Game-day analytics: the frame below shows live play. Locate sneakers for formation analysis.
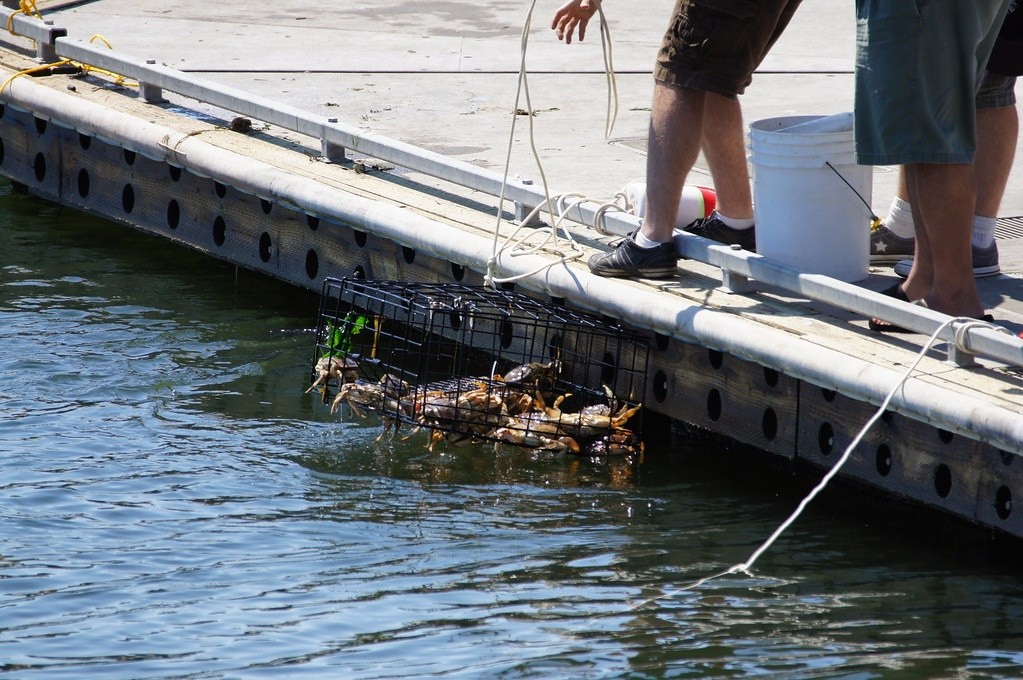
[587,226,678,277]
[869,220,916,264]
[893,239,1001,278]
[683,210,756,252]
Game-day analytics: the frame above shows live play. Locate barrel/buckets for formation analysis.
[748,113,881,283]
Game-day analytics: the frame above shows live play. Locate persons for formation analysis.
[853,0,1023,332]
[551,0,802,275]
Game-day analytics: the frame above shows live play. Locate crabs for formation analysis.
[303,357,646,462]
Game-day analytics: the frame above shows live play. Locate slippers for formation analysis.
[877,282,913,304]
[869,298,995,333]
